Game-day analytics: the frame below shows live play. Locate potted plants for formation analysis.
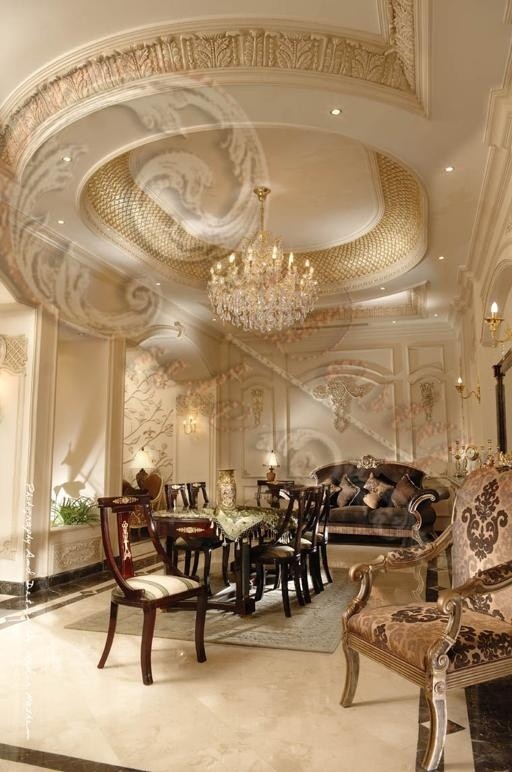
[51,495,103,576]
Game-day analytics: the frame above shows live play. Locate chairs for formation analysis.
[340,455,512,772]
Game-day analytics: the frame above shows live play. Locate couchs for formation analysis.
[297,455,440,545]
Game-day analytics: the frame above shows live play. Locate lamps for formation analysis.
[455,376,480,404]
[206,186,318,334]
[262,450,280,482]
[130,447,155,490]
[483,302,512,348]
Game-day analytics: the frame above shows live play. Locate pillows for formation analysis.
[316,472,421,510]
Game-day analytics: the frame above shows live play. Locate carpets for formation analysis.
[64,563,360,653]
